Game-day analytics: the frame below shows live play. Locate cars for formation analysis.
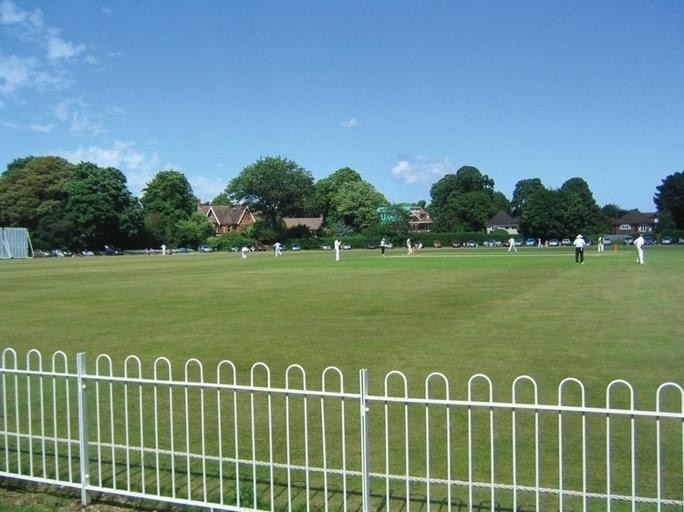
[279,244,286,251]
[290,243,300,251]
[339,242,352,250]
[318,244,332,250]
[197,243,213,253]
[367,241,378,249]
[33,243,125,257]
[414,236,684,249]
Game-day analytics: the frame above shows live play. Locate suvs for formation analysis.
[385,241,393,249]
[254,242,267,251]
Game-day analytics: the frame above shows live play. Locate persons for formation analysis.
[507,238,518,252]
[633,233,645,264]
[147,244,174,256]
[334,239,341,261]
[598,236,604,252]
[537,237,543,248]
[381,238,387,255]
[273,240,282,256]
[406,238,416,256]
[241,245,249,262]
[573,234,586,264]
[545,239,549,247]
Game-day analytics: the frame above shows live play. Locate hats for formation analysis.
[576,234,584,238]
